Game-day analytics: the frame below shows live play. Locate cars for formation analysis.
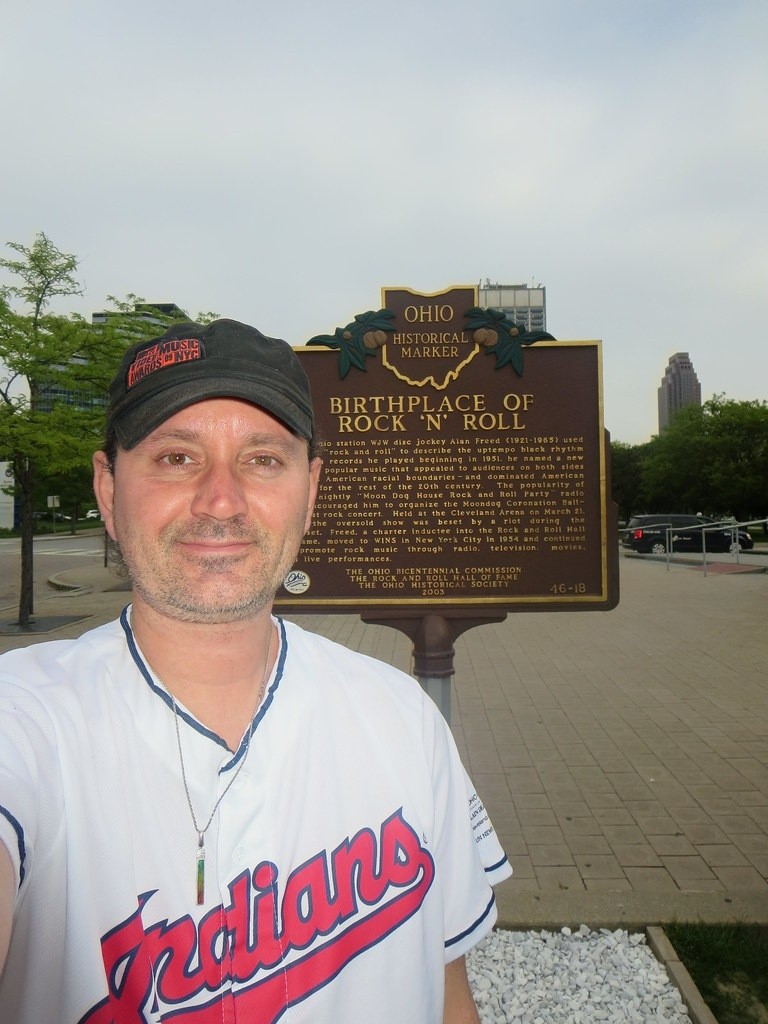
[86,510,104,521]
[32,511,71,521]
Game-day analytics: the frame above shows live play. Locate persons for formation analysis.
[0,317,514,1023]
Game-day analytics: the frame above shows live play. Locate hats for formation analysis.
[105,318,315,451]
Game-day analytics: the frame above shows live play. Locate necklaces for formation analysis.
[145,624,274,905]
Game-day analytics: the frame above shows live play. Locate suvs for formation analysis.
[622,514,754,555]
